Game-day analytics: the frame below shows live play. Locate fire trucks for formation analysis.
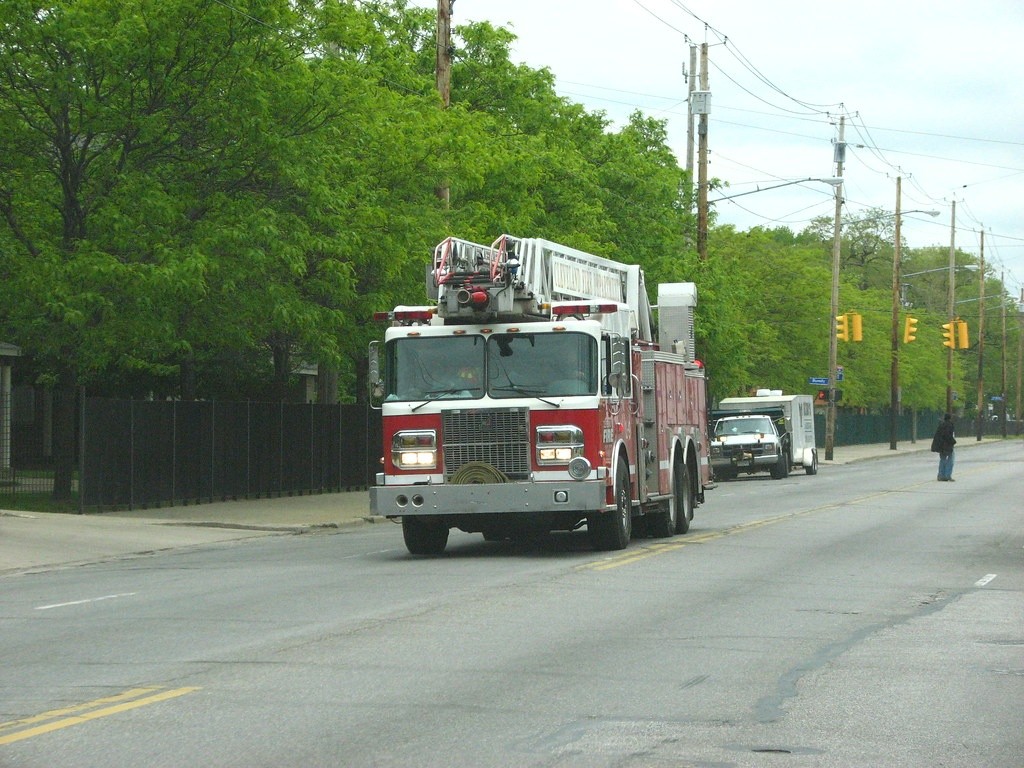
[367,234,717,557]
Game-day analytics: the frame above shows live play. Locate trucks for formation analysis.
[709,388,819,479]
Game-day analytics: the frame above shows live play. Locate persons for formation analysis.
[931,413,956,481]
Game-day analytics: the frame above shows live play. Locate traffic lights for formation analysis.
[904,314,918,344]
[832,389,843,402]
[941,322,955,349]
[817,389,827,400]
[834,313,848,342]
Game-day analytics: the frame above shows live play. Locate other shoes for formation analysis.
[947,476,955,481]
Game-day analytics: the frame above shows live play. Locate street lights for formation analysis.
[889,262,982,450]
[821,206,939,458]
[945,290,1012,413]
[693,176,843,266]
[974,297,1024,441]
